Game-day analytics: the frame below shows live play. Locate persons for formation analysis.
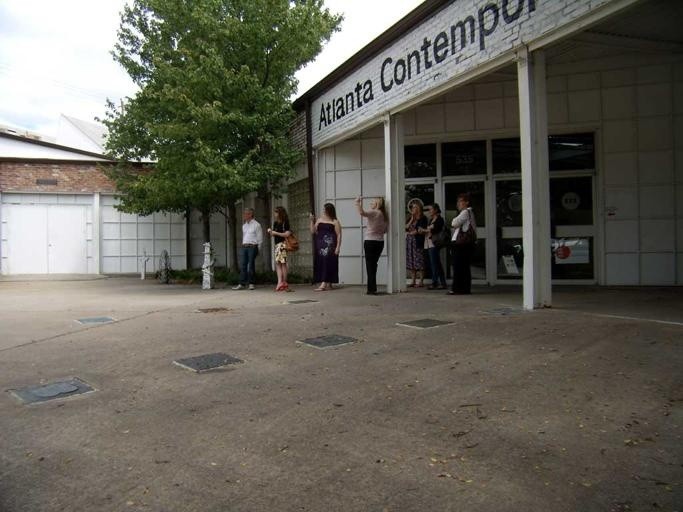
[444,196,477,296]
[307,202,341,293]
[403,200,429,289]
[266,206,292,293]
[416,201,448,291]
[351,191,388,295]
[230,207,263,291]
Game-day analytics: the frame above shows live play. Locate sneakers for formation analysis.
[408,282,469,295]
[232,284,244,290]
[314,283,333,291]
[249,284,255,290]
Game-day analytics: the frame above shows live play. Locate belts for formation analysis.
[243,244,256,248]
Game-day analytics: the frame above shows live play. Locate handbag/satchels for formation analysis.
[285,235,299,251]
[432,225,451,247]
[457,224,476,243]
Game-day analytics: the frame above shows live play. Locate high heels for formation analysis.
[272,284,288,291]
